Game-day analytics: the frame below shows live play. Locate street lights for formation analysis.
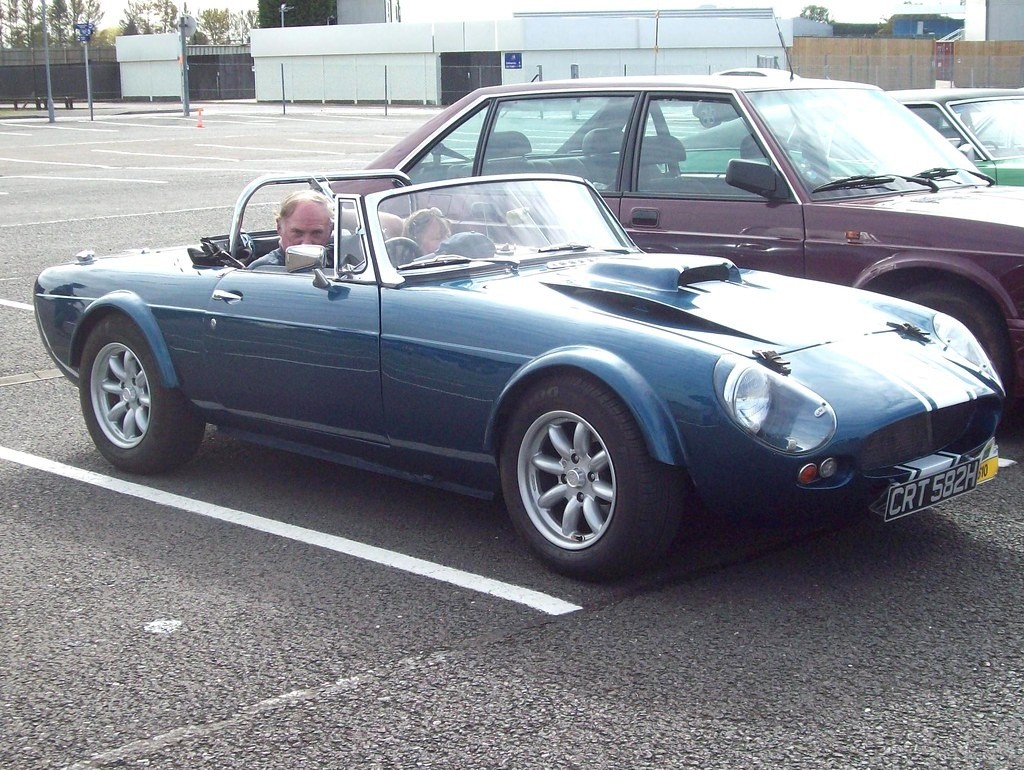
[278,4,294,28]
[327,15,334,25]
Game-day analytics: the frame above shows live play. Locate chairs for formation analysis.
[342,209,403,258]
[636,135,710,195]
[243,236,340,268]
[447,127,661,191]
[741,132,785,160]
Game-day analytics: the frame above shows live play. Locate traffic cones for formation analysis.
[197,108,204,127]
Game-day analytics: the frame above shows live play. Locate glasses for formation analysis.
[412,207,442,226]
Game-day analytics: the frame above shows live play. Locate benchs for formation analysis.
[10,97,76,108]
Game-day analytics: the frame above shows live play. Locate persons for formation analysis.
[397,208,452,266]
[245,189,405,272]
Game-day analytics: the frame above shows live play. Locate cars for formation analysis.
[663,90,1024,191]
[33,168,1018,582]
[328,74,1024,424]
